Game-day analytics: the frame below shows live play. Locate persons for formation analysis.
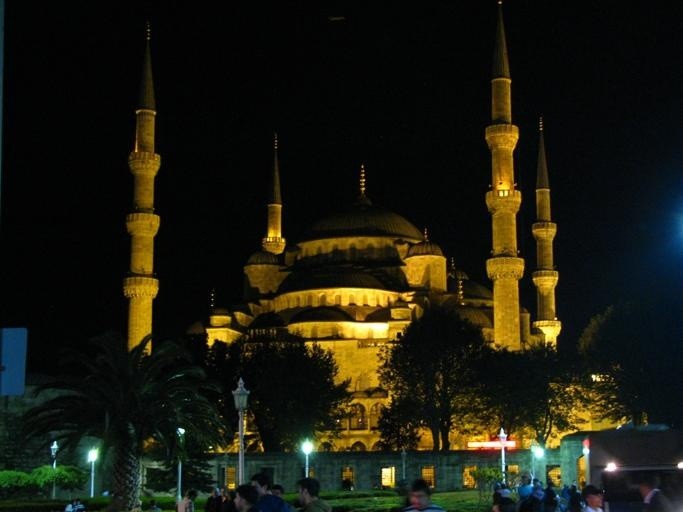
[177,490,197,512]
[297,478,332,512]
[64,498,86,512]
[146,500,161,512]
[205,473,296,512]
[492,474,604,512]
[638,476,675,512]
[402,479,446,512]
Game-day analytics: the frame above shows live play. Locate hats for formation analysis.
[583,485,608,497]
[521,471,532,480]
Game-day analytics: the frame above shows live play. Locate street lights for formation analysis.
[50,440,60,499]
[401,447,408,481]
[497,427,508,484]
[88,447,97,498]
[231,377,251,487]
[302,441,313,480]
[530,444,539,486]
[176,428,186,500]
